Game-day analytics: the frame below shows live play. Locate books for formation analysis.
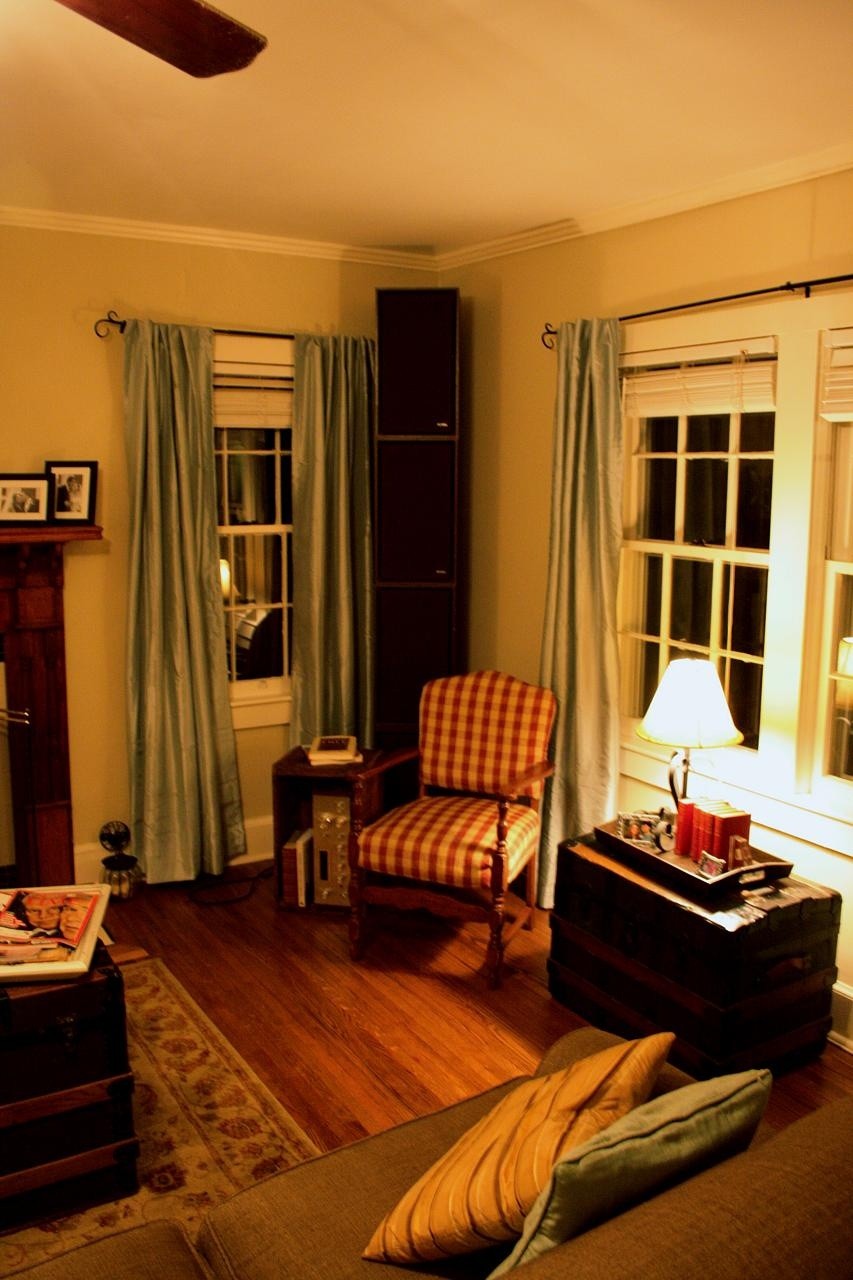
[675,798,751,872]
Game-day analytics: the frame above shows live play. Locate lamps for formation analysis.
[638,657,744,812]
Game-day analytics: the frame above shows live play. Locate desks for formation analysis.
[273,742,381,910]
[0,948,143,1230]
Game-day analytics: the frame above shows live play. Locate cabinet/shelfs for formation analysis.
[548,822,843,1082]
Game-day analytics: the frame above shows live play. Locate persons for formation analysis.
[21,894,93,939]
[56,477,76,512]
[630,822,656,841]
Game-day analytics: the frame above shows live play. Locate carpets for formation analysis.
[0,958,320,1278]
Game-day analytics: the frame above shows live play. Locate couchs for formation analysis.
[16,1028,853,1279]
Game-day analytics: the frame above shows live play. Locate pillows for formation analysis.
[364,1034,684,1264]
[489,1070,768,1280]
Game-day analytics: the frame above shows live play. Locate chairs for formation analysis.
[349,673,556,984]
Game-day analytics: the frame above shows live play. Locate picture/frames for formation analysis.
[0,884,112,984]
[0,459,99,527]
